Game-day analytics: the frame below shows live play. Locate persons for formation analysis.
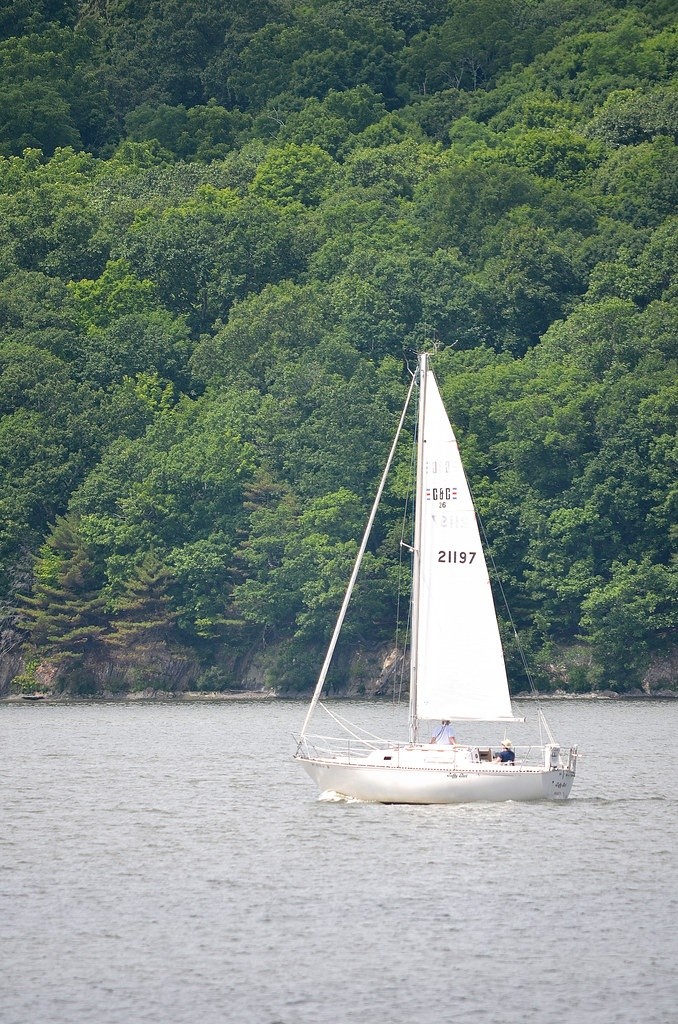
[495,740,515,766]
[430,720,457,745]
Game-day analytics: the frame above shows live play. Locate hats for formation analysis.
[502,739,513,749]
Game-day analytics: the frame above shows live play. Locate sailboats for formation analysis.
[294,352,577,806]
